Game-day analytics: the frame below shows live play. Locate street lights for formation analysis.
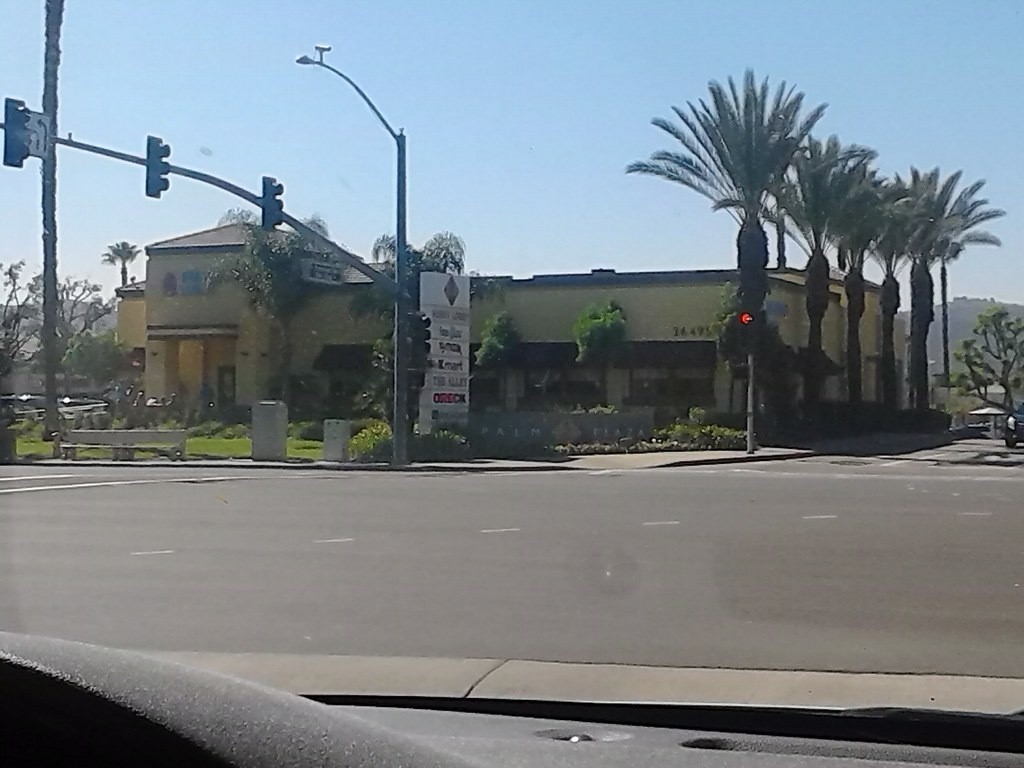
[297,55,407,465]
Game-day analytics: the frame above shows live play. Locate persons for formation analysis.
[1006,410,1020,447]
[31,372,226,439]
[952,410,963,428]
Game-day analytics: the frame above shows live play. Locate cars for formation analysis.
[1005,402,1024,447]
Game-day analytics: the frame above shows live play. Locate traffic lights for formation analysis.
[262,176,283,226]
[146,135,171,197]
[740,312,755,325]
[416,310,432,353]
[3,97,32,168]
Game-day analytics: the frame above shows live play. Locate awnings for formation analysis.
[313,342,376,370]
[761,341,845,378]
[468,340,583,368]
[615,340,721,373]
